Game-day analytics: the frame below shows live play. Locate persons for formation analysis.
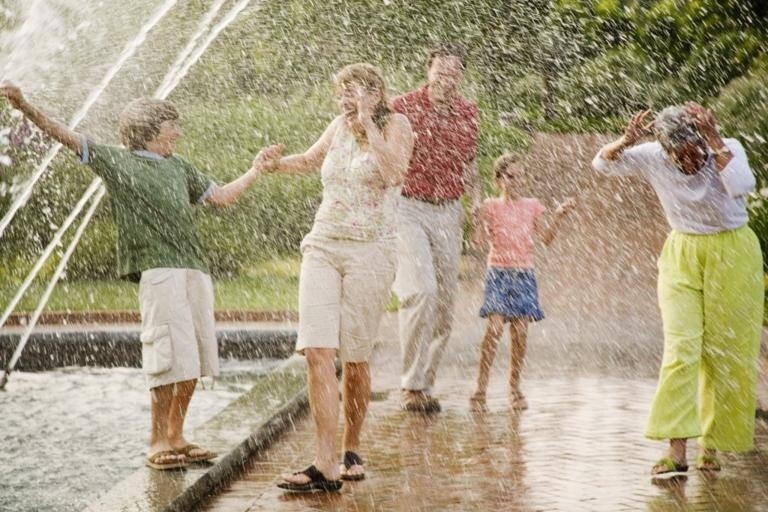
[0,79,288,470]
[468,151,577,416]
[385,46,480,413]
[252,62,414,491]
[591,102,765,483]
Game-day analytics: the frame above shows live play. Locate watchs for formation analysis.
[711,145,728,164]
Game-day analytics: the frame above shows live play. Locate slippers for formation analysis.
[339,451,365,480]
[277,465,343,493]
[650,458,689,479]
[142,444,217,469]
[696,454,721,472]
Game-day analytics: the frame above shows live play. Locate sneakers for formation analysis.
[399,389,440,414]
